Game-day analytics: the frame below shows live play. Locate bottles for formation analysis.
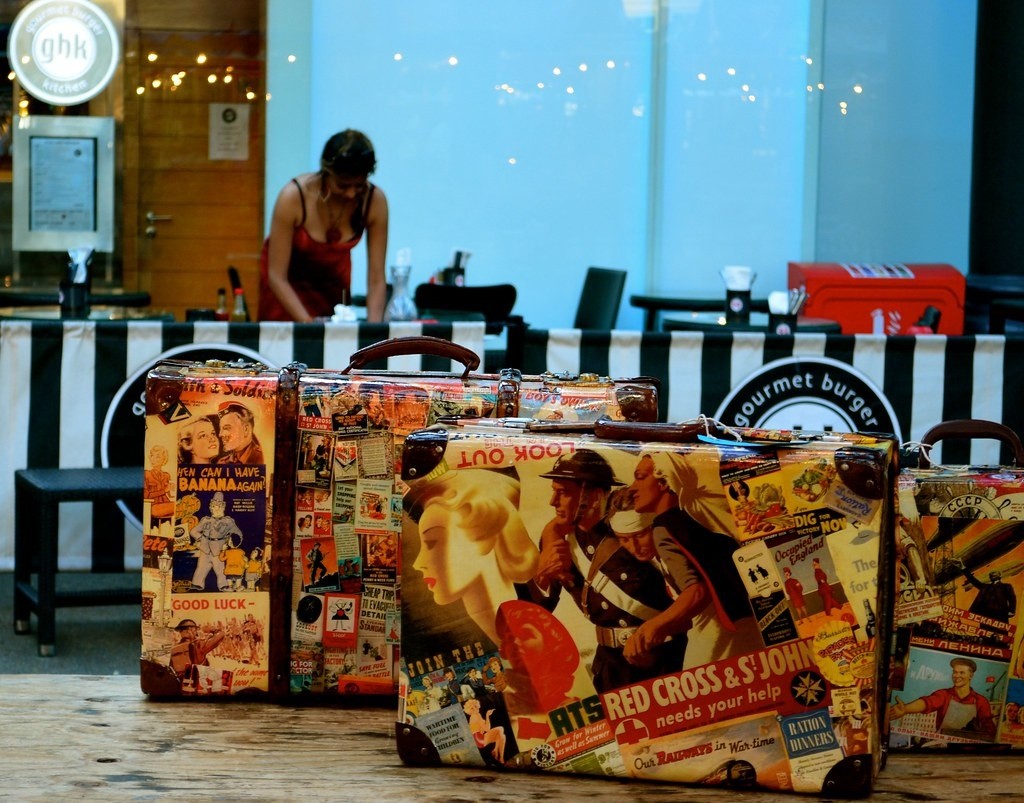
[215,288,230,321]
[233,288,245,322]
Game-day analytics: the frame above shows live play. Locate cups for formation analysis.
[726,288,751,326]
[444,268,464,288]
[769,313,797,334]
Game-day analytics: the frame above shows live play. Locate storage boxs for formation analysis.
[787,262,966,336]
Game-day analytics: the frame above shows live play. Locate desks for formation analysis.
[529,328,1023,466]
[0,318,484,571]
[0,672,1024,803]
[664,314,841,333]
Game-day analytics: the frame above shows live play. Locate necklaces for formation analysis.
[325,190,345,243]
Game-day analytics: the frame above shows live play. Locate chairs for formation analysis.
[572,267,627,329]
[630,295,771,333]
[416,284,517,320]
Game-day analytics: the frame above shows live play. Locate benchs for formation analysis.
[14,468,144,656]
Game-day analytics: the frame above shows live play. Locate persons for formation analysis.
[256,130,389,323]
